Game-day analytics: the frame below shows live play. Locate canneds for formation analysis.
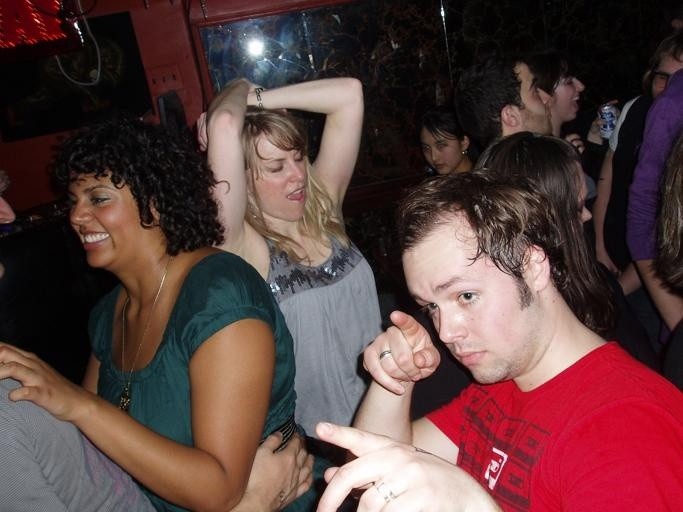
[598,105,619,138]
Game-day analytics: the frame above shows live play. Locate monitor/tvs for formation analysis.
[0,10,155,143]
